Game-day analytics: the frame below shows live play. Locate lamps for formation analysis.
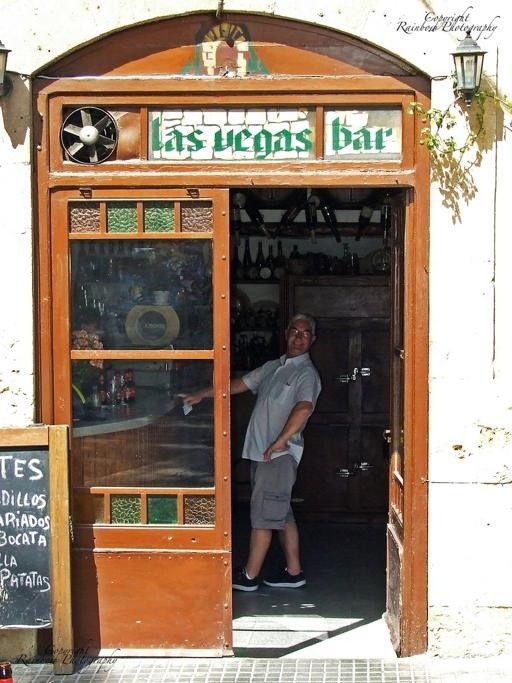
[449,27,488,109]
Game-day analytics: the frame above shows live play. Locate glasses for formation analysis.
[288,328,312,338]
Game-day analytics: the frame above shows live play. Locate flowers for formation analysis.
[70,329,107,387]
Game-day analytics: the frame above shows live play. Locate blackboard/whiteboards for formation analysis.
[0,424,72,629]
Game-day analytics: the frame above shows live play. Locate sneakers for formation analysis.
[263,568,307,588]
[233,567,259,592]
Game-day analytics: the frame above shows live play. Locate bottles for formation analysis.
[77,240,130,282]
[230,203,243,247]
[380,203,391,245]
[90,368,136,410]
[272,201,303,240]
[232,238,300,281]
[321,205,342,243]
[304,200,318,245]
[244,204,272,240]
[355,203,376,242]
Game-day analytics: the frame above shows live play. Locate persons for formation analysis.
[72,305,116,393]
[175,312,324,592]
